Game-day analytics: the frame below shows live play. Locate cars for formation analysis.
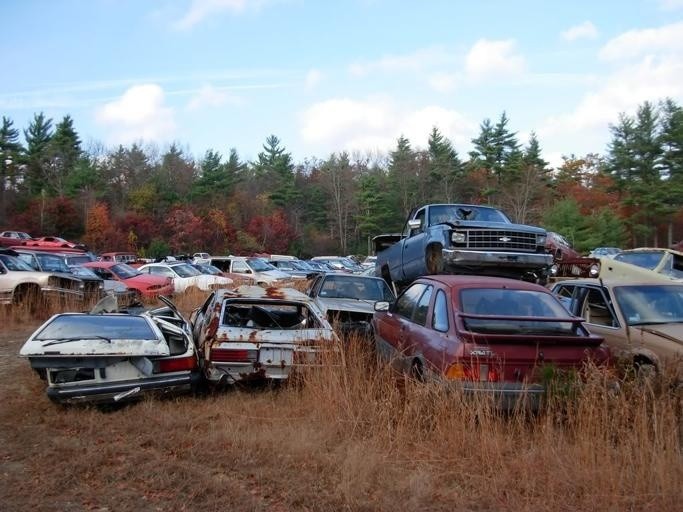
[550,276,683,397]
[370,271,620,418]
[309,271,398,345]
[597,245,683,282]
[588,247,624,258]
[189,283,346,389]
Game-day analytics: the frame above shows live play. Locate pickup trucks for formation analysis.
[373,203,555,292]
